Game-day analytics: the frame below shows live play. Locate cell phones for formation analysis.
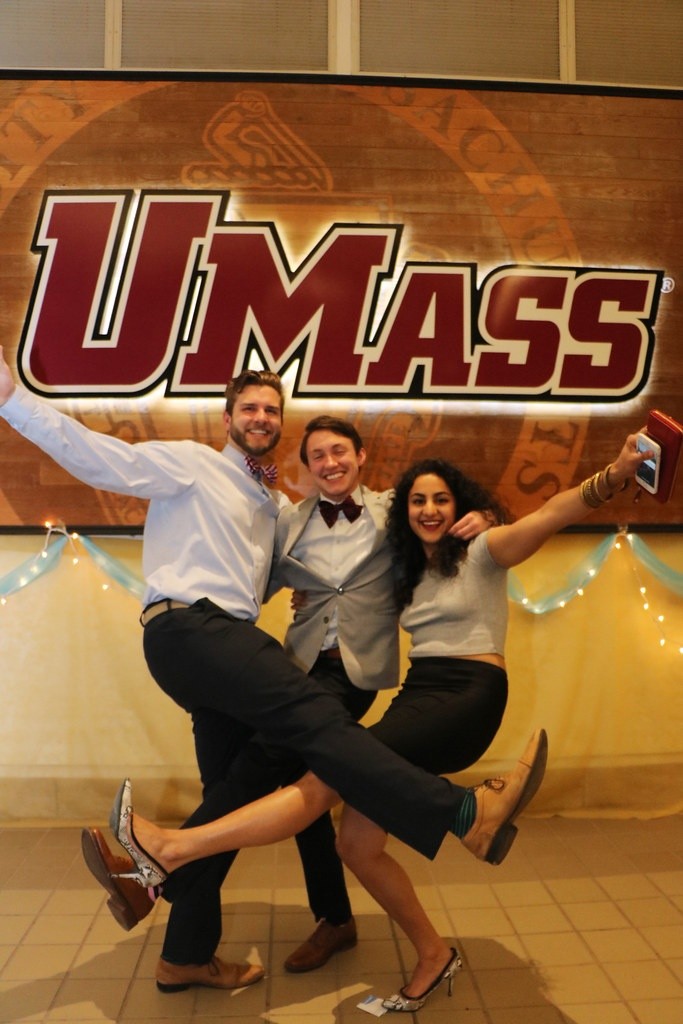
[635,433,661,494]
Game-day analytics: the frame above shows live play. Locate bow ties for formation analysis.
[319,495,363,529]
[247,455,277,484]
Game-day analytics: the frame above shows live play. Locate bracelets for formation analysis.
[579,463,630,511]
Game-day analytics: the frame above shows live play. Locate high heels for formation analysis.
[382,947,463,1012]
[108,777,168,889]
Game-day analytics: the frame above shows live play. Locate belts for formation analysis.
[142,600,190,626]
[319,646,342,659]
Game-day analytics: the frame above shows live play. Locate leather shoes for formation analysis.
[461,727,548,865]
[82,826,155,931]
[284,916,357,972]
[156,955,265,994]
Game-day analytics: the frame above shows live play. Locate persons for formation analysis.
[0,346,548,994]
[110,426,654,1010]
